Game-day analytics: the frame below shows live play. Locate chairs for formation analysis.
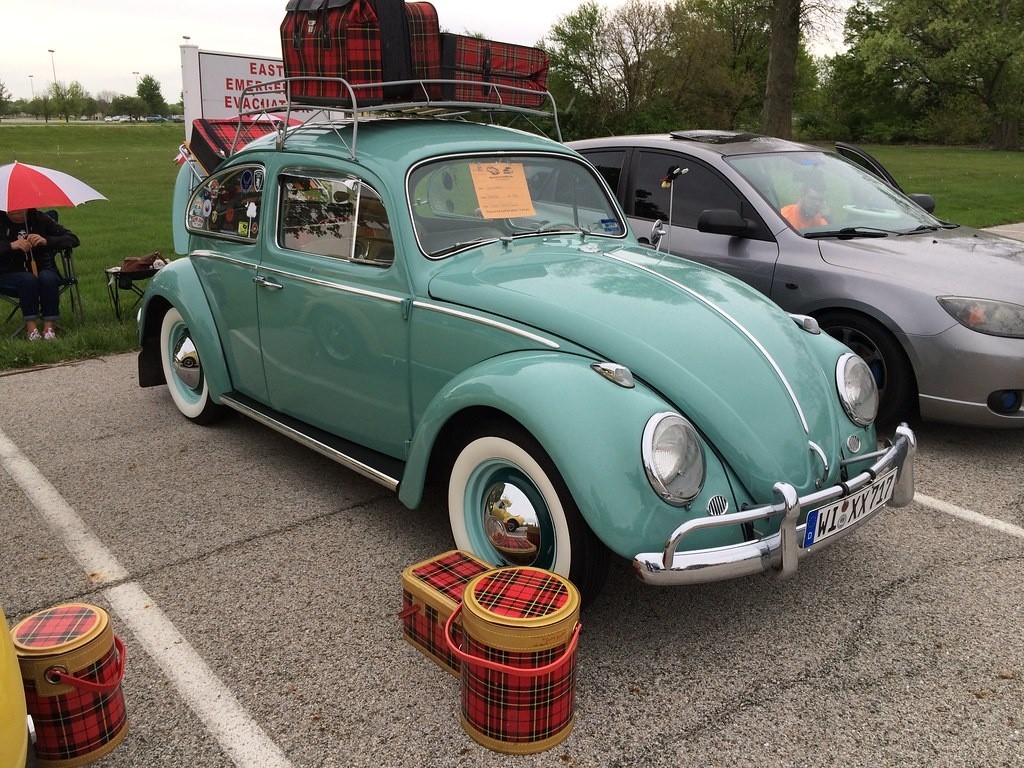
[0,210,83,338]
[422,228,506,256]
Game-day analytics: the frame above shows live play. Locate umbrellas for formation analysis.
[0,159,110,277]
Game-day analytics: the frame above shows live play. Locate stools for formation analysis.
[104,258,170,326]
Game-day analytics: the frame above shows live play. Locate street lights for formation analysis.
[28,75,36,100]
[132,72,140,88]
[48,49,57,85]
[183,35,190,45]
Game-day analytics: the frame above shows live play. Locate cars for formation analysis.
[133,76,916,614]
[476,129,1024,433]
[112,115,185,123]
[104,116,112,122]
[80,116,88,121]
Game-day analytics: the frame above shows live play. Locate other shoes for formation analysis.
[42,328,56,340]
[27,328,42,340]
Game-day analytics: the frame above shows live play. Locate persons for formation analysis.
[780,178,831,229]
[0,208,81,341]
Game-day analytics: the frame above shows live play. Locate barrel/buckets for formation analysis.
[444,565,581,755]
[11,602,130,768]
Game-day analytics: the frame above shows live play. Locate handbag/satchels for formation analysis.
[189,117,289,173]
[279,0,443,107]
[439,30,549,105]
[120,252,168,272]
